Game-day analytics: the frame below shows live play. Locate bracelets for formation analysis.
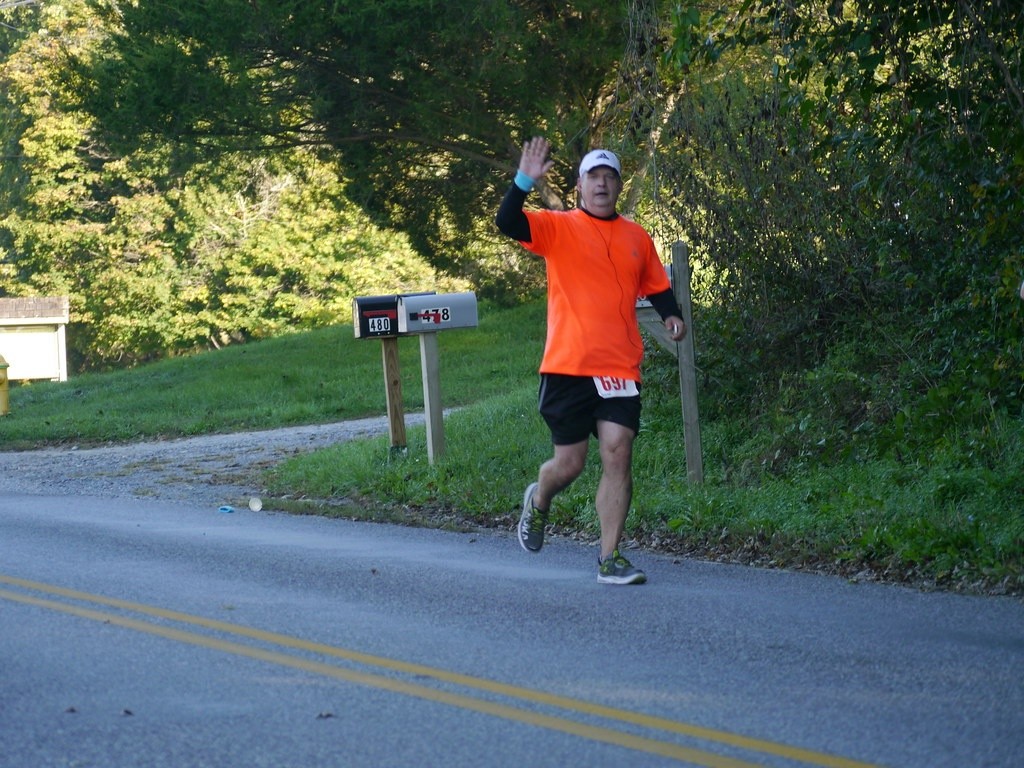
[514,170,538,194]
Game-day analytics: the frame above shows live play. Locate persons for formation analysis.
[495,137,687,585]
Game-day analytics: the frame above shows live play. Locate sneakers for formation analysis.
[597,549,647,585]
[518,482,551,553]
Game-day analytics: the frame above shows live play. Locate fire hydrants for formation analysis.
[0,352,10,416]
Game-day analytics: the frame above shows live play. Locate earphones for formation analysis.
[579,183,581,188]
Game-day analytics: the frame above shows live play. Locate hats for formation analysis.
[579,149,622,181]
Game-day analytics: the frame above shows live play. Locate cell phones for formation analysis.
[674,324,678,335]
